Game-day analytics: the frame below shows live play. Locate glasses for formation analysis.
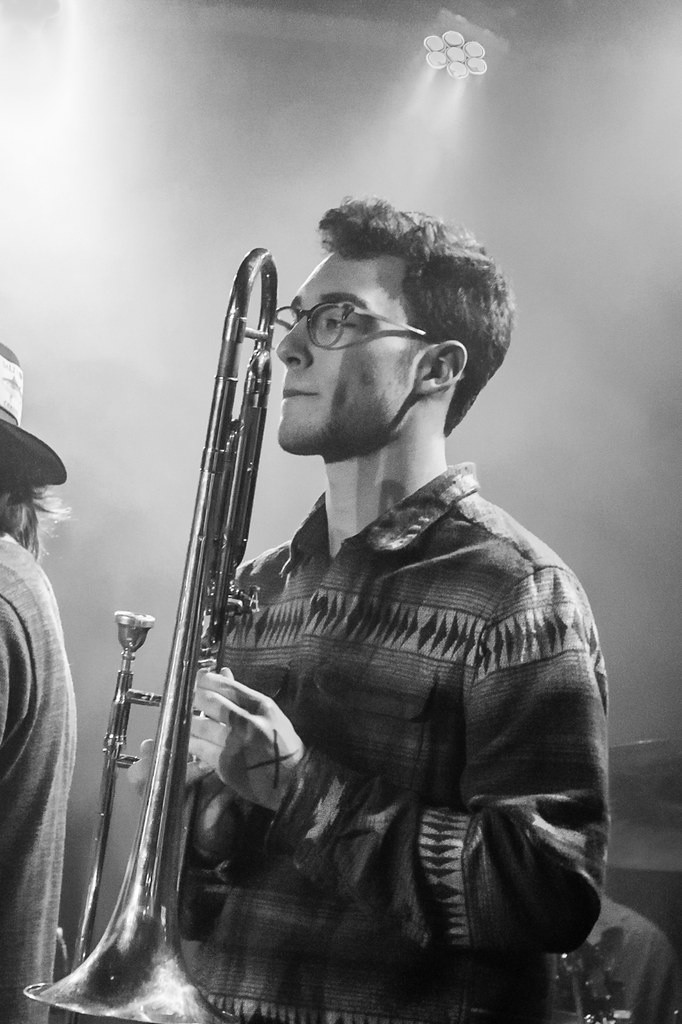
[267,302,434,351]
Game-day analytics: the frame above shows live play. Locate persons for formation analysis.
[0,419,69,1023]
[127,208,609,1023]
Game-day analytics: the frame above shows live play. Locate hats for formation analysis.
[0,341,68,488]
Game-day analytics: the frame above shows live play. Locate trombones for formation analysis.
[22,245,278,1024]
